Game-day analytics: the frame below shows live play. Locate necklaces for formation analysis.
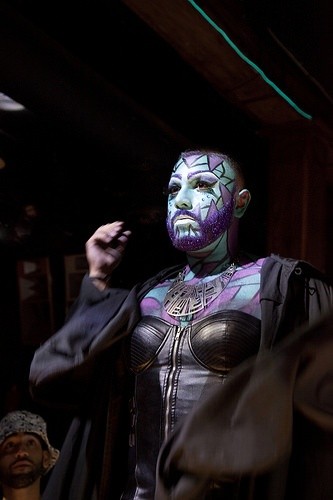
[163,260,237,318]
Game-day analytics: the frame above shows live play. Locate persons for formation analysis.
[29,149,333,498]
[0,412,62,500]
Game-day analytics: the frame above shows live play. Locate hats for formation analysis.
[0,410,58,476]
